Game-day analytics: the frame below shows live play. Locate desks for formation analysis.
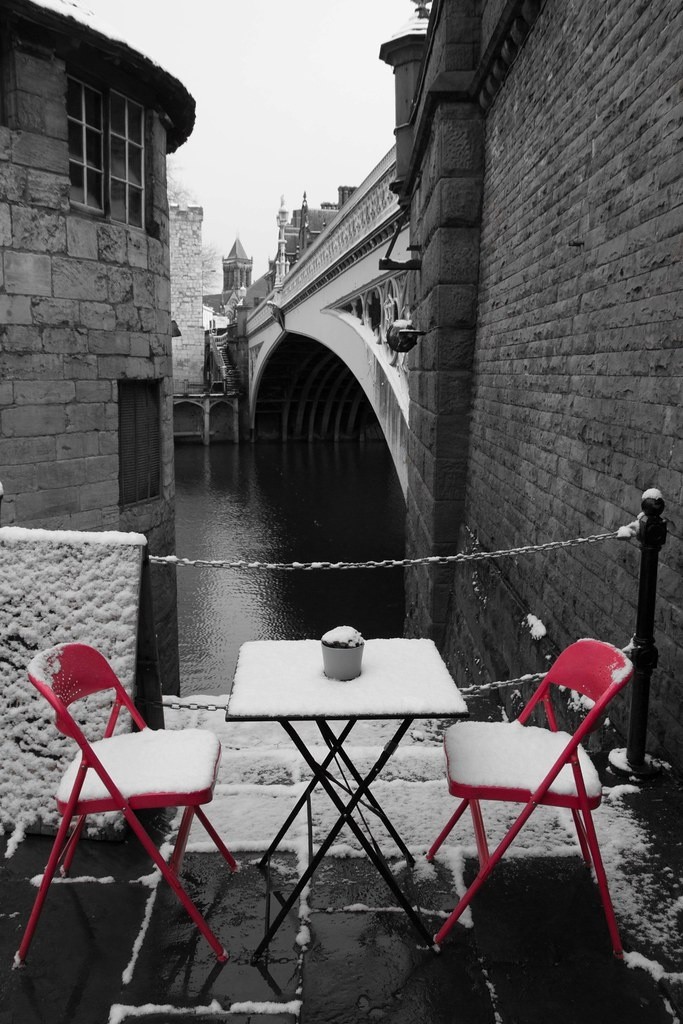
[225,639,468,966]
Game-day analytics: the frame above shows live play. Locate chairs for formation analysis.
[426,638,635,961]
[12,643,241,967]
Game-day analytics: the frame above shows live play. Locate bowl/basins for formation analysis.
[322,642,363,679]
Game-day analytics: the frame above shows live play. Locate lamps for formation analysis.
[387,324,428,353]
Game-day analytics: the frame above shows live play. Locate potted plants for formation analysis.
[321,625,365,682]
